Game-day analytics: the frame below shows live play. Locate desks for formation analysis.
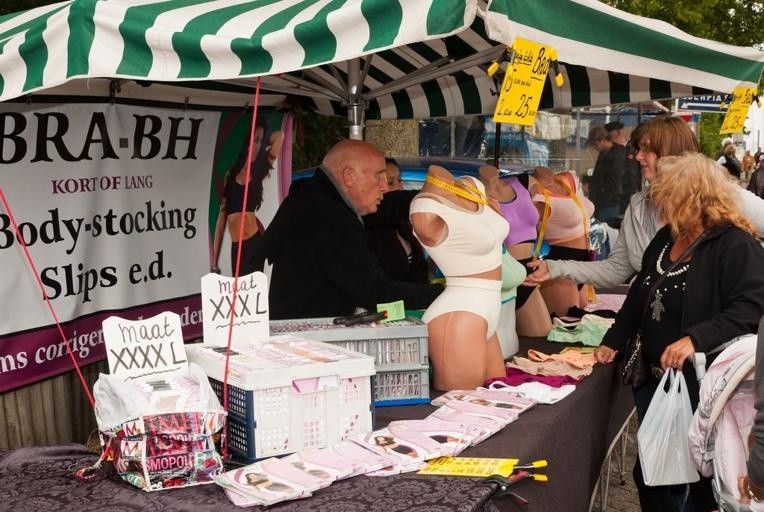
[1,278,640,512]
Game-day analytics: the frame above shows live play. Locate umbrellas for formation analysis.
[0,0,764,141]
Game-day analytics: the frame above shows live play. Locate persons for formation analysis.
[376,435,419,460]
[598,153,764,512]
[471,398,522,411]
[717,147,741,180]
[381,158,429,282]
[714,141,732,160]
[606,122,625,143]
[246,472,292,495]
[410,166,508,389]
[753,147,763,161]
[523,115,699,287]
[526,166,592,311]
[214,118,285,276]
[739,295,764,511]
[743,150,755,178]
[588,127,625,226]
[432,435,467,445]
[748,162,764,199]
[480,163,551,336]
[262,140,446,319]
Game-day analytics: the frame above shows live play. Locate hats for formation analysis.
[606,121,622,130]
[582,127,610,147]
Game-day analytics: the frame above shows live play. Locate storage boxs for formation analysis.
[184,335,374,471]
[269,318,432,407]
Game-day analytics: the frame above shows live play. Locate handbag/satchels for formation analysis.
[622,228,713,388]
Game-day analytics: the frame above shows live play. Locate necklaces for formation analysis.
[656,239,690,277]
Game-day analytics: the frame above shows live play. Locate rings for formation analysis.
[671,360,677,365]
